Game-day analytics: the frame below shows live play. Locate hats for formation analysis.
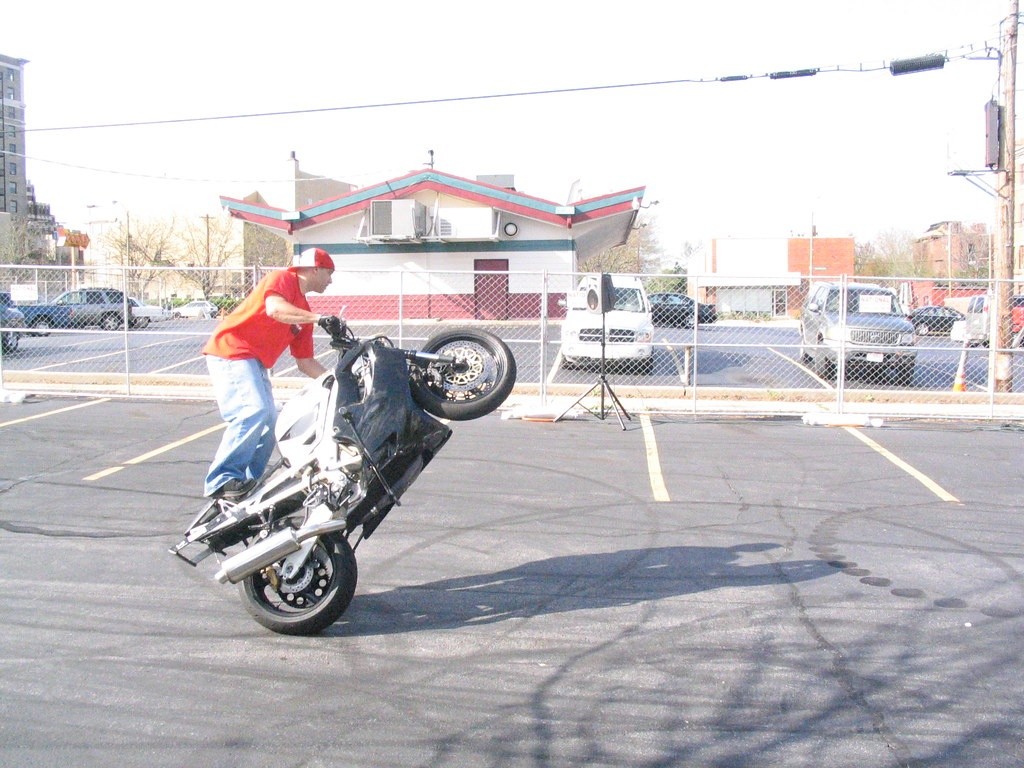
[287,247,335,270]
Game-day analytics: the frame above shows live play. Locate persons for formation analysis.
[202,248,347,498]
[1012,298,1024,348]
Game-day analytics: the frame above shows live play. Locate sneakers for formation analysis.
[209,478,257,498]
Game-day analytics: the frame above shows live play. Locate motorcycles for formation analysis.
[168,302,518,635]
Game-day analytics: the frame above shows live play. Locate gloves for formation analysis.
[318,315,342,335]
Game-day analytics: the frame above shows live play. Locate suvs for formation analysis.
[53,287,132,331]
[798,281,915,382]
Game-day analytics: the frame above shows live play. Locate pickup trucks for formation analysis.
[3,292,74,337]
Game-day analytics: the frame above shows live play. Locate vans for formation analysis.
[965,294,1024,349]
[559,273,654,376]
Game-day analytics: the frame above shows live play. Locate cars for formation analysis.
[647,294,717,329]
[128,298,169,329]
[0,303,24,356]
[171,300,218,319]
[907,306,966,336]
